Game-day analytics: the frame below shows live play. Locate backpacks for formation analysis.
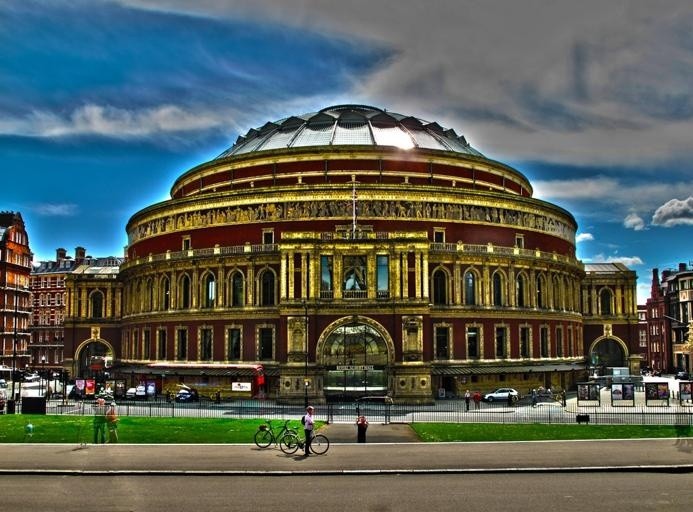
[301,416,305,425]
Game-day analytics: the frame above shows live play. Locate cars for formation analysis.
[0,377,8,410]
[24,373,40,382]
[71,381,200,404]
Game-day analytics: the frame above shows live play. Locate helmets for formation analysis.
[306,406,314,410]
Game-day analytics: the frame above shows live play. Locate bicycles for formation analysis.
[279,423,329,456]
[253,419,298,448]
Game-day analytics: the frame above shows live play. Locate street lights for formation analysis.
[52,372,59,398]
[297,297,310,407]
[10,284,29,400]
[40,354,44,395]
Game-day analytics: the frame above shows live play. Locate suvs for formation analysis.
[674,371,689,380]
[336,395,395,416]
[481,386,520,402]
